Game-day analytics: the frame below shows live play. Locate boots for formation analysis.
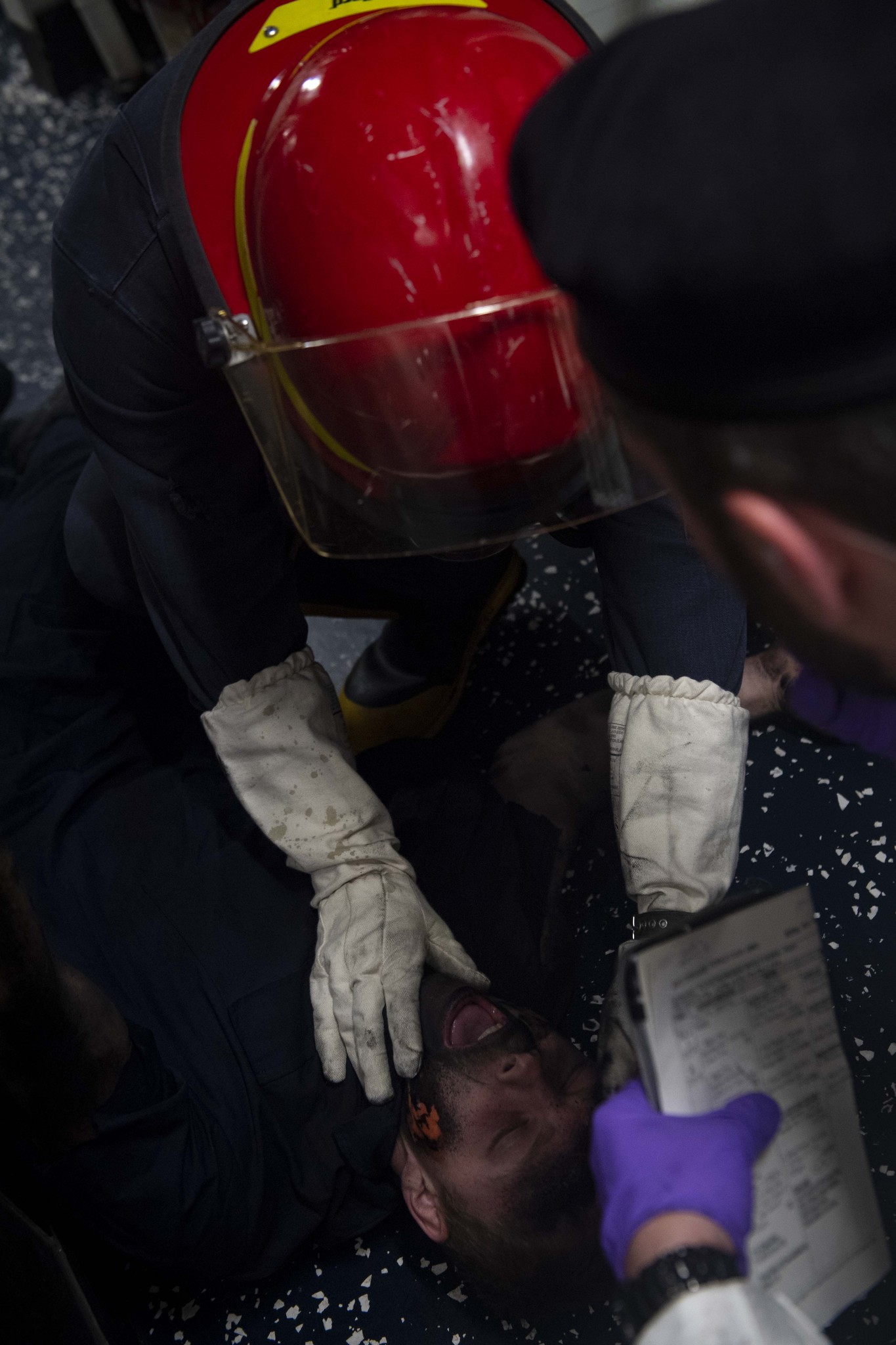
[300,553,531,757]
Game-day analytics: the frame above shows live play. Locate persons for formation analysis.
[505,0,895,1345]
[1,367,605,1344]
[45,0,750,1097]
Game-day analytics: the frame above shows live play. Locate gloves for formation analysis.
[198,643,494,1104]
[786,669,896,763]
[590,1075,780,1280]
[602,669,752,1096]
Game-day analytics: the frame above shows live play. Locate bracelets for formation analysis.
[608,1247,746,1344]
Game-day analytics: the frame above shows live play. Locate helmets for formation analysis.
[159,1,665,561]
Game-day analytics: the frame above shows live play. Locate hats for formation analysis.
[506,2,896,423]
